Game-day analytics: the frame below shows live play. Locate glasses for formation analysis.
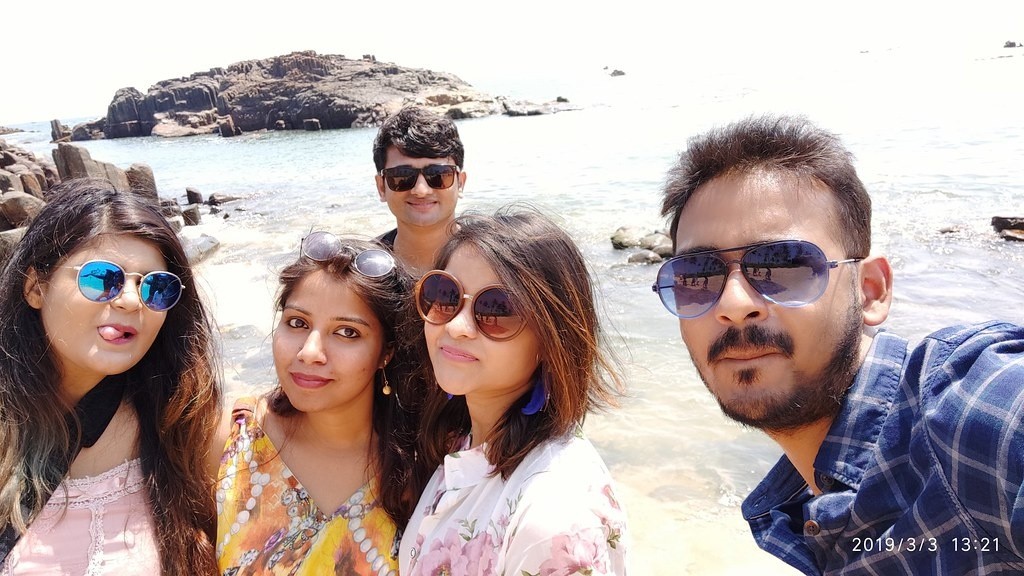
[48,260,186,313]
[415,270,534,342]
[301,231,398,278]
[651,239,862,318]
[378,165,459,192]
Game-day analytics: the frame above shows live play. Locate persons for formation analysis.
[653,112,1024,576]
[1,178,225,576]
[396,201,636,576]
[366,104,469,526]
[213,231,457,575]
